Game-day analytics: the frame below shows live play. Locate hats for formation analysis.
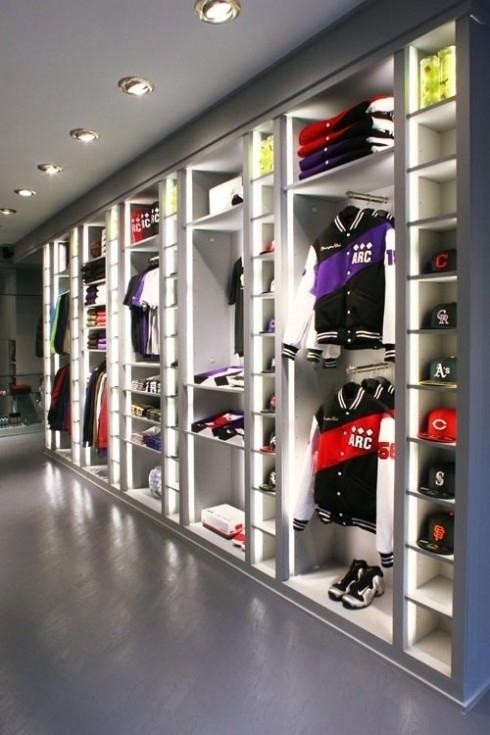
[419,407,457,443]
[418,512,453,554]
[259,240,277,491]
[420,303,456,330]
[420,358,457,387]
[419,463,454,499]
[423,251,455,274]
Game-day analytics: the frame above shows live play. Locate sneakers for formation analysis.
[342,567,384,610]
[329,559,367,601]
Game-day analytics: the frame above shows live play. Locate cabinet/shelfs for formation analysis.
[42,5,483,709]
